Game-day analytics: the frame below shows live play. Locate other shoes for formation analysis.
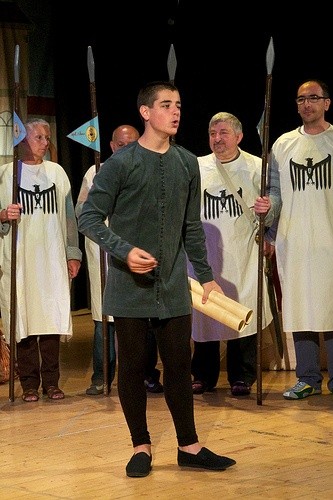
[125,452,152,477]
[177,446,236,470]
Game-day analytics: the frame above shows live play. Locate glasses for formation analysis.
[296,96,329,105]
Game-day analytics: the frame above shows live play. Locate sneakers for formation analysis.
[145,381,160,394]
[192,380,210,394]
[231,381,252,396]
[283,381,322,399]
[86,379,110,394]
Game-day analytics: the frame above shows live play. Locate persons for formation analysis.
[183,112,268,397]
[79,82,236,477]
[255,80,333,400]
[75,125,163,395]
[0,117,82,402]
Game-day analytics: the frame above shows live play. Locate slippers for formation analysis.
[22,388,40,402]
[46,386,65,399]
